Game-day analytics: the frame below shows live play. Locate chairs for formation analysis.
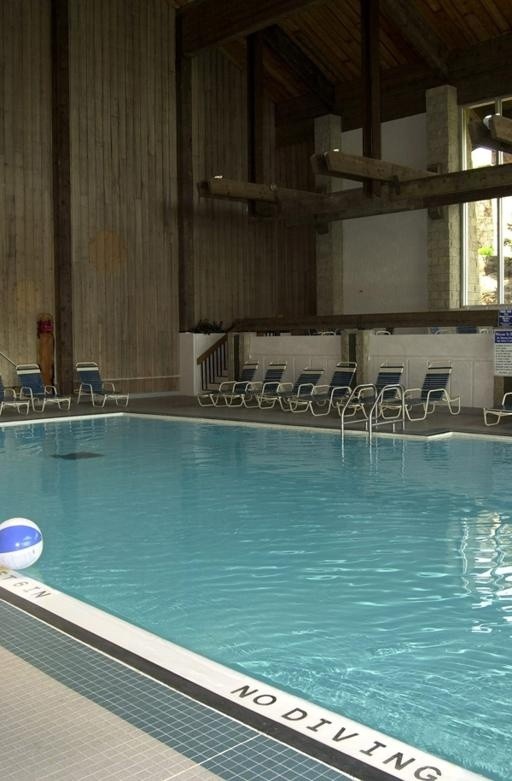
[196,357,464,428]
[74,361,132,410]
[15,362,73,414]
[0,373,30,419]
[481,391,512,428]
